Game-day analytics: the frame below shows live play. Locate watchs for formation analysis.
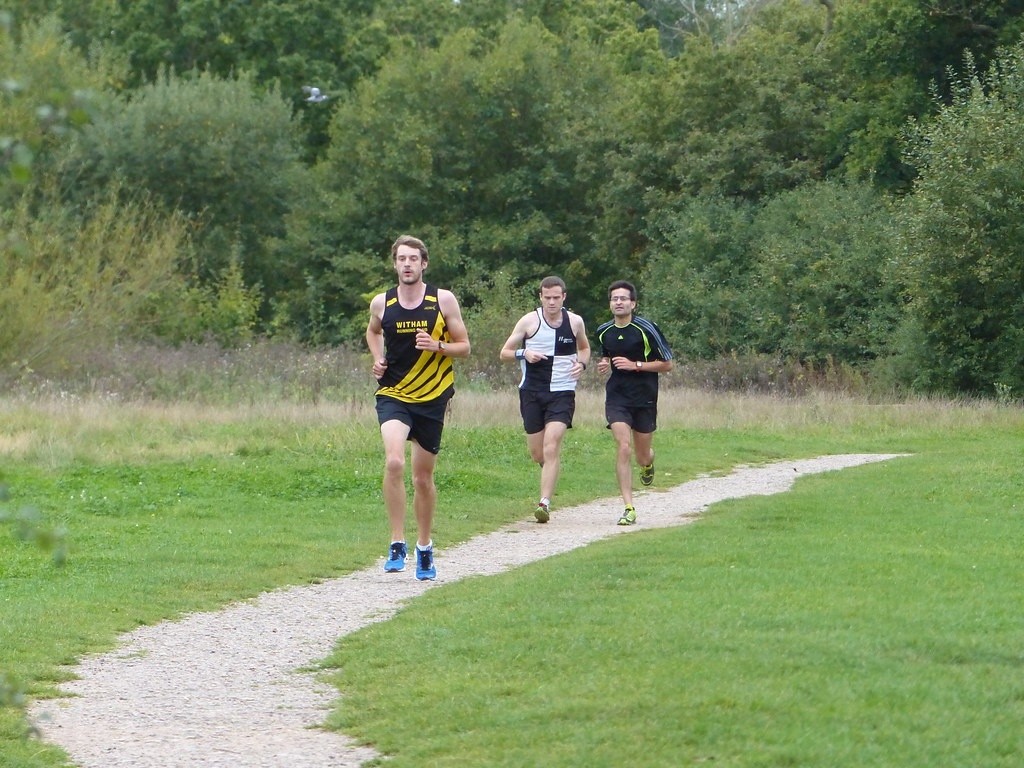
[439,340,447,355]
[636,361,643,371]
[578,362,586,371]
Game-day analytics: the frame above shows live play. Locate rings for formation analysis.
[601,369,604,373]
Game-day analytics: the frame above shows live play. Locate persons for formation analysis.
[363,233,471,581]
[595,280,674,526]
[499,275,591,522]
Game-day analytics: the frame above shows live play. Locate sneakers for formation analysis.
[535,504,549,523]
[617,505,636,525]
[384,542,409,572]
[415,548,437,580]
[640,464,654,486]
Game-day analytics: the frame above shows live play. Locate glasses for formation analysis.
[609,296,631,301]
